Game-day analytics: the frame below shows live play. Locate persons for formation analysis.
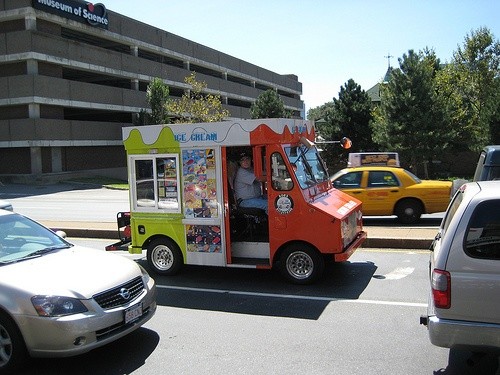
[234,151,287,218]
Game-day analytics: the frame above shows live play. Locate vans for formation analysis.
[474,145,500,181]
[420,181,499,349]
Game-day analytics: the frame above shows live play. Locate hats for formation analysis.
[237,151,249,160]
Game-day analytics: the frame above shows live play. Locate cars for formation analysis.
[0,204,159,375]
[329,166,453,224]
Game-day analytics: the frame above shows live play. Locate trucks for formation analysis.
[106,116,368,286]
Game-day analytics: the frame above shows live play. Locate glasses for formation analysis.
[240,156,249,161]
